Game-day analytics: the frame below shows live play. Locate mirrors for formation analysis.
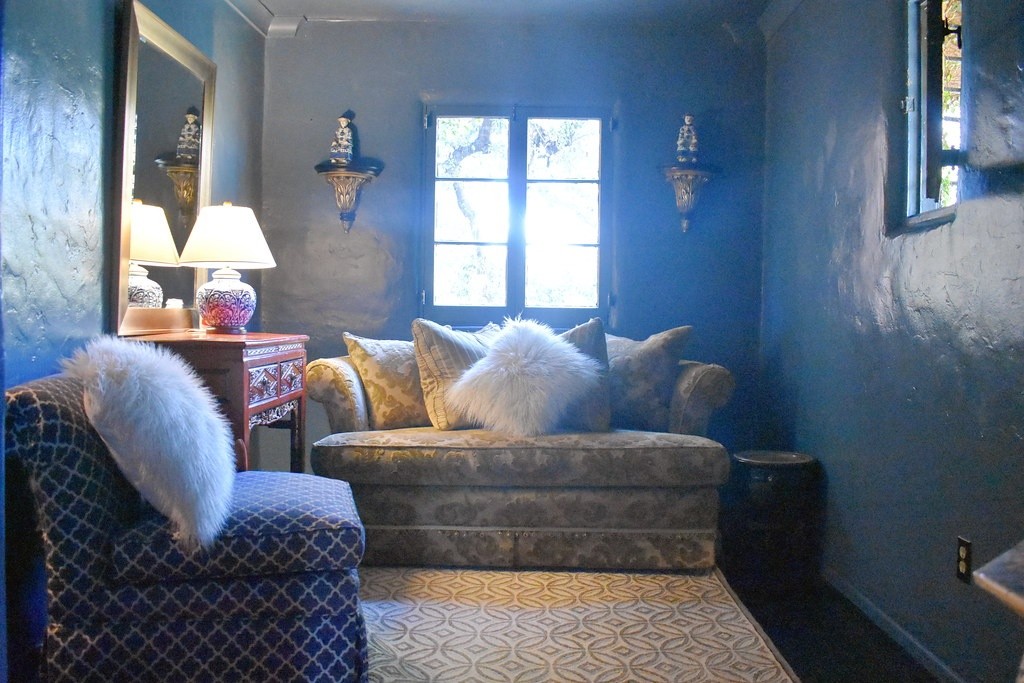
[116,0,217,337]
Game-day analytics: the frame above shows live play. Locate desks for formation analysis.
[128,333,311,472]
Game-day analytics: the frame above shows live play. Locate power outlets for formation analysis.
[956,535,971,583]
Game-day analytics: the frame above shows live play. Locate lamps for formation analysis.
[311,162,385,233]
[129,198,182,311]
[660,162,723,233]
[154,157,203,229]
[179,200,277,334]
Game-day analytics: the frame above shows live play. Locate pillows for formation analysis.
[604,326,692,431]
[344,332,435,431]
[558,316,611,436]
[412,318,501,431]
[80,331,238,554]
[445,316,601,436]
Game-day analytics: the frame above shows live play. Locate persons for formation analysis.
[176,112,199,161]
[329,117,353,163]
[677,114,697,162]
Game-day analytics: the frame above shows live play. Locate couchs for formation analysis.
[305,354,734,568]
[5,371,367,683]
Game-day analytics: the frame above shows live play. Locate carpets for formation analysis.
[360,570,802,683]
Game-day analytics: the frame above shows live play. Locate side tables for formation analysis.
[737,450,815,574]
